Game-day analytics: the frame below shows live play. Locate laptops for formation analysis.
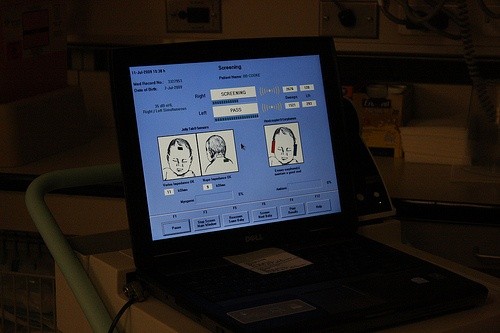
[108,35,489,333]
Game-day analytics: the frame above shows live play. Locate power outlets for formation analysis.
[398,4,472,35]
[481,5,500,36]
[319,2,379,38]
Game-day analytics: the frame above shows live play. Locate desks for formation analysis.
[0,127,500,270]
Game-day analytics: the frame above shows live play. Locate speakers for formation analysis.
[341,97,396,222]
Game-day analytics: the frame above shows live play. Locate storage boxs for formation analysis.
[353,86,414,127]
